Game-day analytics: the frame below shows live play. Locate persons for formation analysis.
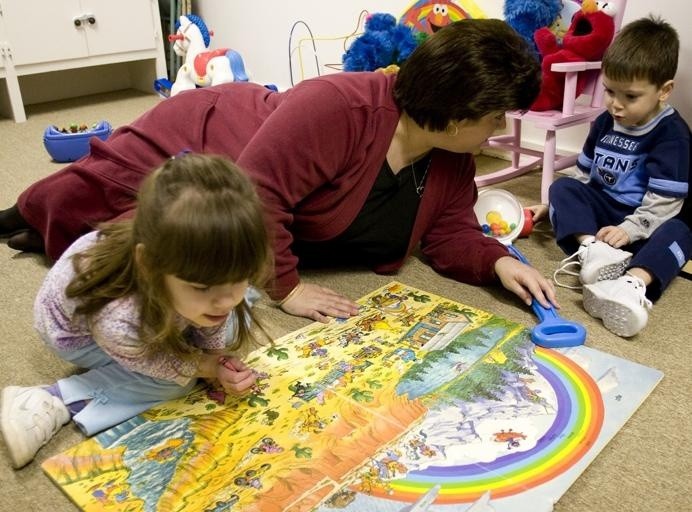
[0,18,563,323]
[2,151,275,471]
[524,11,691,337]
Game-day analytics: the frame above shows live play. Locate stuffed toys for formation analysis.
[532,0,618,111]
[339,10,418,73]
[502,0,563,57]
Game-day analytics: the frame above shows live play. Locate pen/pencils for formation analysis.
[218,356,260,394]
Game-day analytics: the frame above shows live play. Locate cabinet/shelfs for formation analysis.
[1,1,171,122]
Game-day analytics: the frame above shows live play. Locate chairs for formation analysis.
[473,1,628,212]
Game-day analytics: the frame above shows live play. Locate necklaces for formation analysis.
[404,120,435,195]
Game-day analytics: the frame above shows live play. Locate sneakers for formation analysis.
[582,275,652,338]
[1,385,70,469]
[553,238,633,290]
